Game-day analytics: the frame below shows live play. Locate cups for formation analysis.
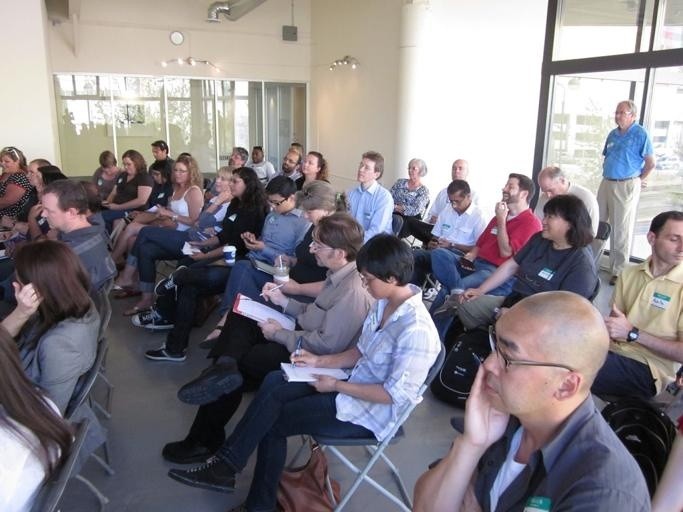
[223,246,237,264]
[273,256,290,280]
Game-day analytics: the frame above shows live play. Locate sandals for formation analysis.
[111,282,134,290]
[123,306,150,316]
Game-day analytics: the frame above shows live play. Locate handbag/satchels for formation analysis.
[275,443,341,512]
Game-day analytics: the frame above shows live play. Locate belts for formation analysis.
[604,175,639,182]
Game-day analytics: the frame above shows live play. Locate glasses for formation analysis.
[407,167,419,171]
[445,197,467,205]
[311,233,335,253]
[3,147,20,160]
[357,271,383,284]
[266,197,288,206]
[488,324,573,373]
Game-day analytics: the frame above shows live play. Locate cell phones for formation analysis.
[458,257,476,269]
[244,237,257,244]
[432,239,439,244]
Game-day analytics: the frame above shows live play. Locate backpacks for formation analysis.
[430,328,492,409]
[601,397,677,500]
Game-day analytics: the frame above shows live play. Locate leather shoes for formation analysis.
[198,325,225,350]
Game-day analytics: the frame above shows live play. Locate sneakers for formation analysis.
[144,318,175,330]
[167,455,237,496]
[432,289,464,318]
[143,342,188,362]
[153,265,187,296]
[131,306,162,327]
[161,430,226,464]
[177,362,244,407]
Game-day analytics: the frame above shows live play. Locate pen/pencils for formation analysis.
[258,283,284,296]
[291,336,303,369]
[279,255,284,273]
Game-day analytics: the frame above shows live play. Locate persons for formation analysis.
[0,139,683,512]
[596,99,656,286]
